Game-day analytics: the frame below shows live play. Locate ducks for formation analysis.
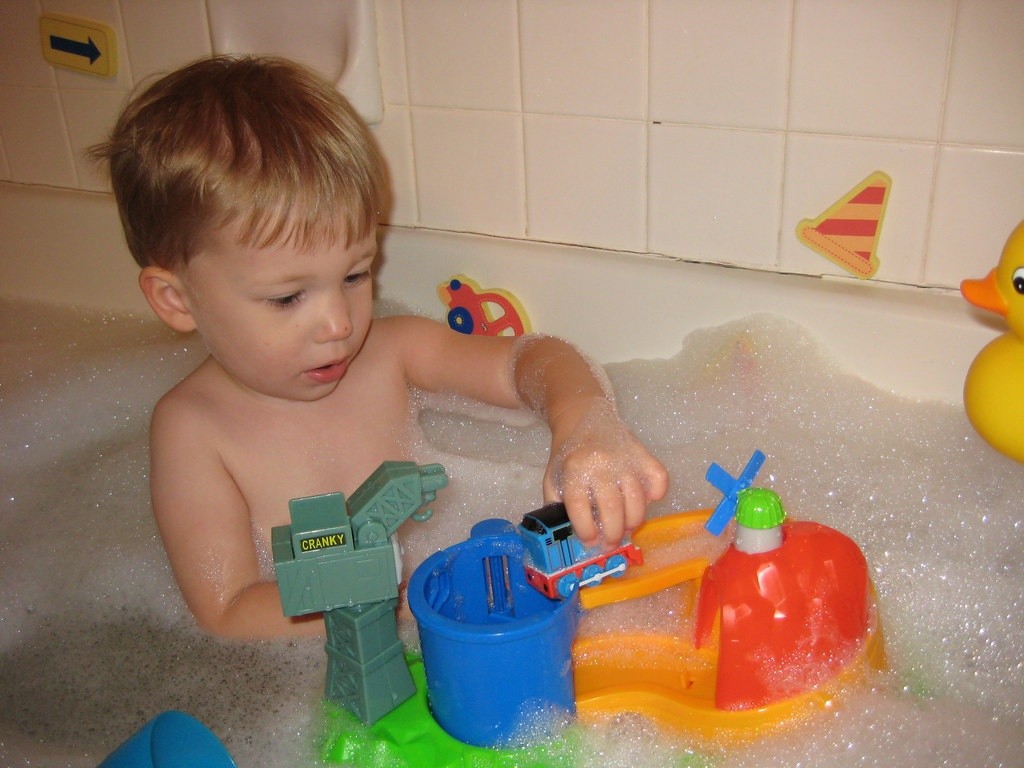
[960,218,1024,464]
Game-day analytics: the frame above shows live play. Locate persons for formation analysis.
[111,55,668,648]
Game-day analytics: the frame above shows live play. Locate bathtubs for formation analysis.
[0,179,1024,768]
